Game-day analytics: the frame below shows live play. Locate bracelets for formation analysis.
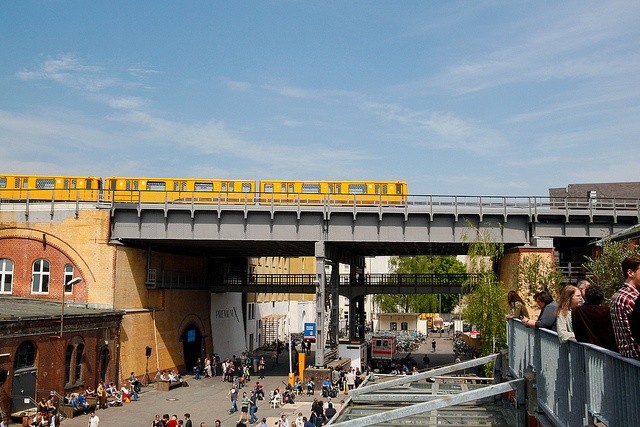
[527,319,529,322]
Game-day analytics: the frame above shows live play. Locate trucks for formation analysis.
[371,337,396,361]
[304,323,316,343]
[432,317,444,333]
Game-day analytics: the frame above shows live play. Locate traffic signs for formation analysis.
[304,323,316,339]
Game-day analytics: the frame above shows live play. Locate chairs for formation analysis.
[294,384,304,397]
[122,389,133,403]
[128,379,139,392]
[269,396,281,409]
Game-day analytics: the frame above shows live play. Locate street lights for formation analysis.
[51,276,82,340]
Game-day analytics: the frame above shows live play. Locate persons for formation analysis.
[63,380,123,409]
[120,383,139,400]
[226,381,268,427]
[556,285,583,344]
[610,255,640,360]
[155,370,189,387]
[194,358,202,380]
[0,415,12,427]
[577,280,592,304]
[88,411,100,427]
[423,354,430,372]
[367,324,370,333]
[274,395,344,426]
[571,284,619,353]
[452,331,483,377]
[431,338,437,353]
[269,376,315,405]
[521,290,559,331]
[128,371,147,394]
[261,336,311,368]
[504,290,530,320]
[200,422,206,427]
[151,413,192,427]
[321,365,375,396]
[29,393,61,426]
[203,350,265,384]
[215,420,224,427]
[366,351,417,374]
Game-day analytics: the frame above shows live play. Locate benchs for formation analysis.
[157,371,186,390]
[62,394,97,421]
